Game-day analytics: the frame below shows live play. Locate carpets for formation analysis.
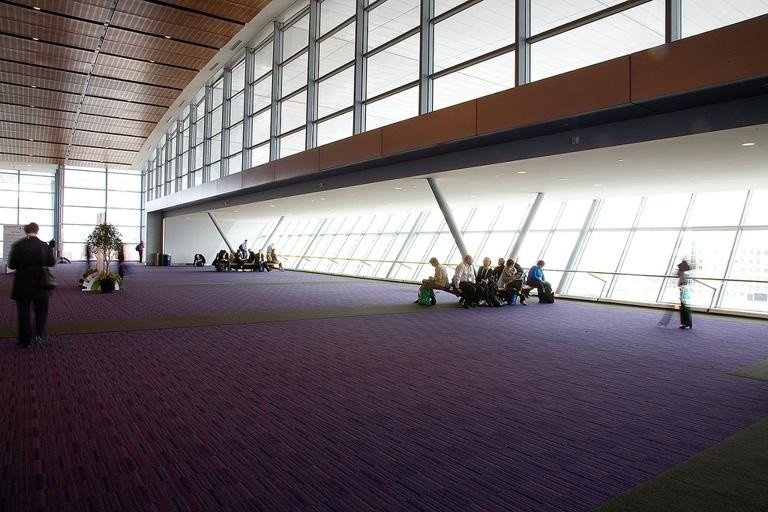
[1,298,767,512]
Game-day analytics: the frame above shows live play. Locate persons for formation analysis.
[48,238,55,248]
[7,222,55,350]
[677,258,693,330]
[413,254,553,309]
[212,238,286,272]
[137,240,144,263]
[191,253,206,267]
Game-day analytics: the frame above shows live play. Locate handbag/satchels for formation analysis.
[41,267,57,289]
[494,296,508,306]
[417,287,430,305]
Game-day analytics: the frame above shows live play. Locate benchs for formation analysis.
[438,282,535,303]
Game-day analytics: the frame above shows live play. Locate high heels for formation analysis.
[680,324,692,329]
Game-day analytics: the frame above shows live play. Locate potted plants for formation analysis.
[87,222,125,292]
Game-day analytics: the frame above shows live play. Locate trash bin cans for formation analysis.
[154,254,171,266]
